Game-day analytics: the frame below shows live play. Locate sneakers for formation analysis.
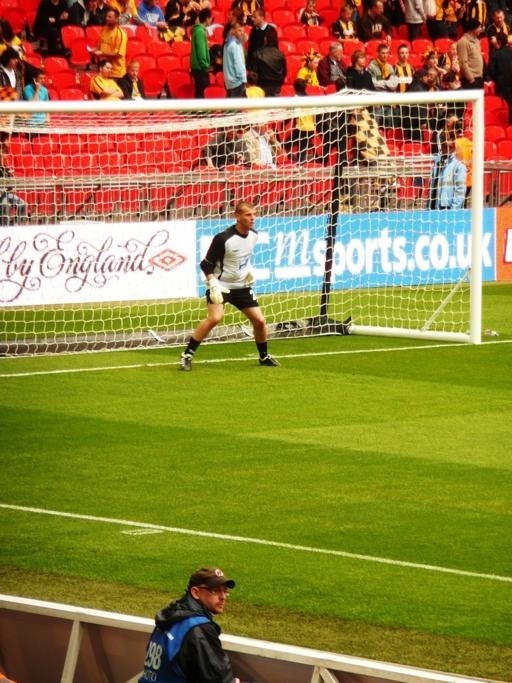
[259,354,279,366]
[180,352,193,371]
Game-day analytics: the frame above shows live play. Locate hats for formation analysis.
[189,568,236,590]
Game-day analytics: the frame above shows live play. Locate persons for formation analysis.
[1,0,213,144]
[189,1,512,216]
[142,568,246,683]
[1,169,29,227]
[179,203,283,372]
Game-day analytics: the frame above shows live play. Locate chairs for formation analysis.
[0,0,511,215]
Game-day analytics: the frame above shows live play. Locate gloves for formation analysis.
[206,278,229,305]
[245,272,256,286]
